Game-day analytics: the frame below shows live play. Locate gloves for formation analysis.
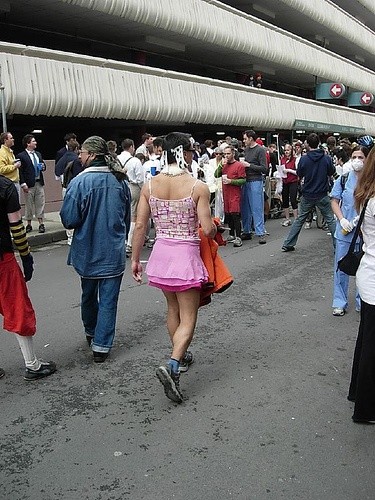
[22,254,34,282]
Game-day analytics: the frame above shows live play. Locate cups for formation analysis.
[151,166,156,175]
[38,163,43,170]
[221,175,228,183]
[239,157,245,163]
[14,159,20,165]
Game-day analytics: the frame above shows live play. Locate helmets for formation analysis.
[358,135,374,148]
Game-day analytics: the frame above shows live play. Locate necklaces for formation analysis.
[161,172,187,177]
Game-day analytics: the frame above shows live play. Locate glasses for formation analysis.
[80,151,94,155]
[215,153,222,155]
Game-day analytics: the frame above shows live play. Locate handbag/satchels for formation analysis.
[336,250,365,277]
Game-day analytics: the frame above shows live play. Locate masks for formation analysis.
[350,159,364,172]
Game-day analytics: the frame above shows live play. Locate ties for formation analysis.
[32,153,39,177]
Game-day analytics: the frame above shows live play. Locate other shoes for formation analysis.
[39,224,44,233]
[281,246,295,252]
[93,351,107,362]
[333,307,345,316]
[233,237,243,247]
[68,237,73,245]
[282,220,291,226]
[125,245,132,253]
[259,236,266,244]
[313,216,317,221]
[226,236,235,243]
[240,233,251,240]
[26,225,32,232]
[305,222,311,229]
[86,336,94,347]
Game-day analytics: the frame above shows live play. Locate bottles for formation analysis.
[341,215,361,236]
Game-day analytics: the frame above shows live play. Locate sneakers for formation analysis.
[156,364,184,404]
[0,367,6,379]
[23,357,57,381]
[178,351,194,371]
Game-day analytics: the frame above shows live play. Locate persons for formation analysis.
[0,131,375,403]
[348,146,375,423]
